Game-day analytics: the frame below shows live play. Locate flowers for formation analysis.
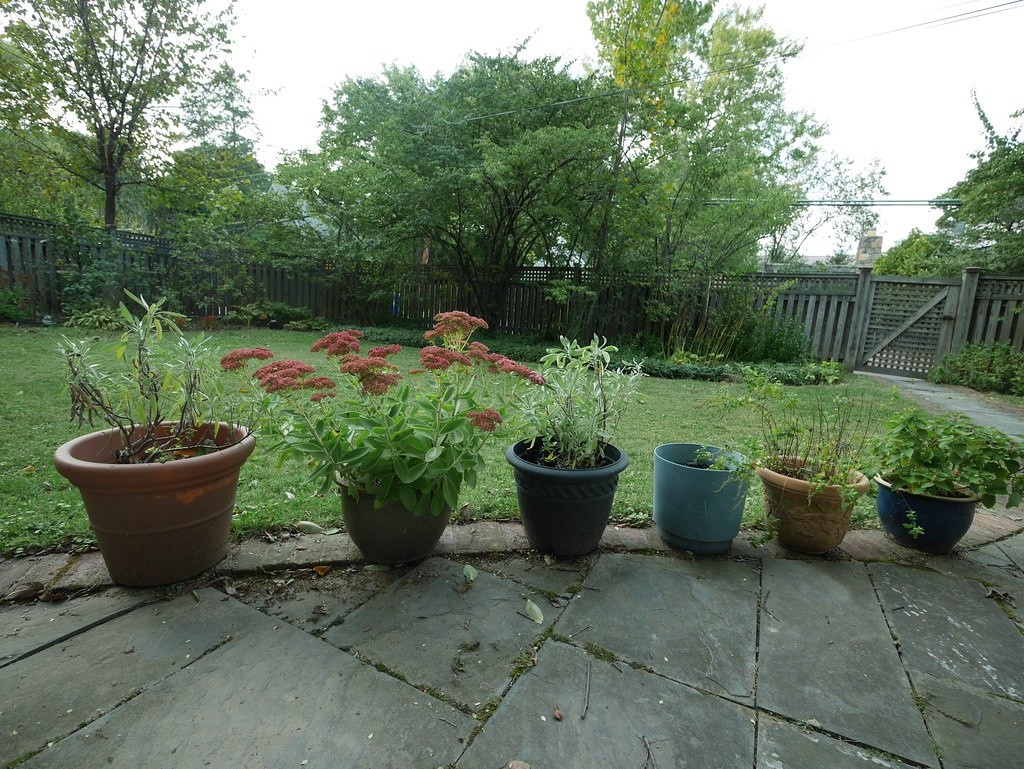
[218,308,550,437]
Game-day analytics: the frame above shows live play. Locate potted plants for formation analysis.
[54,289,1024,588]
